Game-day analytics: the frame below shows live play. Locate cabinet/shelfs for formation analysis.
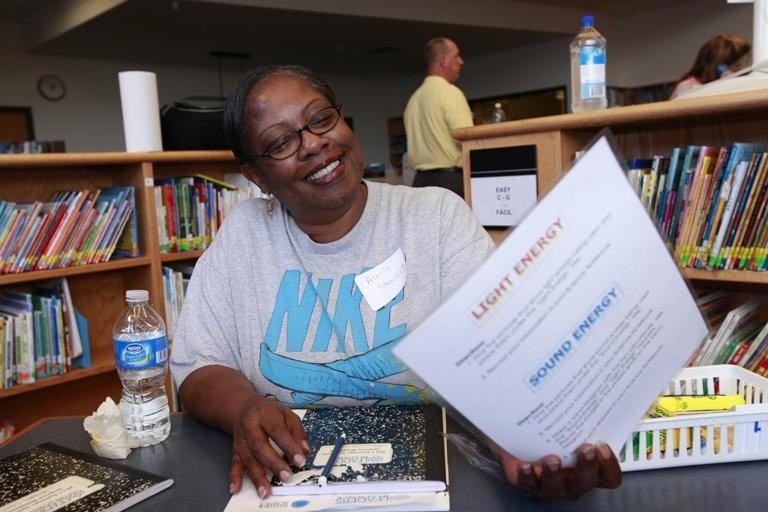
[454,91,768,382]
[0,152,276,438]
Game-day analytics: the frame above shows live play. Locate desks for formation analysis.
[0,416,768,512]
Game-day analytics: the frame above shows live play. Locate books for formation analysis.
[1,277,84,390]
[223,407,451,512]
[683,286,768,379]
[1,442,174,512]
[161,263,194,341]
[575,138,767,273]
[1,185,140,274]
[270,404,447,497]
[153,173,262,255]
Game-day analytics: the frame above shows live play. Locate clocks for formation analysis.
[38,75,65,101]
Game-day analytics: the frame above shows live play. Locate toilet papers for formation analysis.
[118,69,165,156]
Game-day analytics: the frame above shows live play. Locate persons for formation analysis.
[668,35,751,100]
[169,64,623,511]
[403,37,475,201]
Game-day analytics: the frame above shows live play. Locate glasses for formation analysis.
[243,104,347,161]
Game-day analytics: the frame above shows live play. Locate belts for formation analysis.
[414,166,464,174]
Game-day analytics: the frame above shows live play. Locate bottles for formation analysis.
[568,15,608,113]
[113,288,171,448]
[492,102,505,123]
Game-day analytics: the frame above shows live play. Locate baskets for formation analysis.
[620,364,767,473]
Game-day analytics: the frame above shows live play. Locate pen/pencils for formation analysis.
[318,431,347,487]
